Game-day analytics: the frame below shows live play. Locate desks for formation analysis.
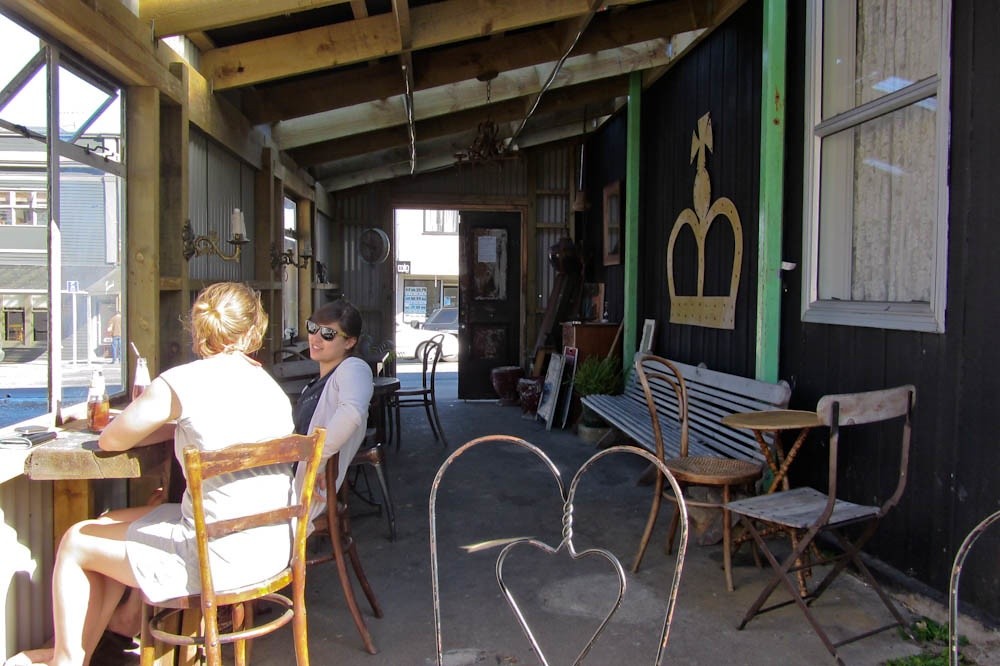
[279,378,401,513]
[271,351,391,442]
[721,409,825,598]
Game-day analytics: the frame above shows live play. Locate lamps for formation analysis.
[455,68,521,180]
[182,208,250,264]
[270,240,312,271]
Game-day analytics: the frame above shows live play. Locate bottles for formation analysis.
[132,358,151,401]
[87,372,110,432]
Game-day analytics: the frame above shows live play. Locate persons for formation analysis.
[0,282,296,666]
[107,311,121,364]
[291,299,374,548]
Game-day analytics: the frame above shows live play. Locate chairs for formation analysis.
[140,334,916,666]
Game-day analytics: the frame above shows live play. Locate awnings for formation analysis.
[84,267,121,294]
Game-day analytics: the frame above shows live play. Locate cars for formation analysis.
[395,318,459,363]
[421,306,458,335]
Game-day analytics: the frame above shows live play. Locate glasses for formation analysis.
[306,320,350,341]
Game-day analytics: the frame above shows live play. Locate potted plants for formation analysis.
[563,352,632,445]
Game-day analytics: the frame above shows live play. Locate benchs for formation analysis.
[579,353,792,498]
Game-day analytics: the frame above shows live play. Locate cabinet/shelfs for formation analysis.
[559,323,621,366]
